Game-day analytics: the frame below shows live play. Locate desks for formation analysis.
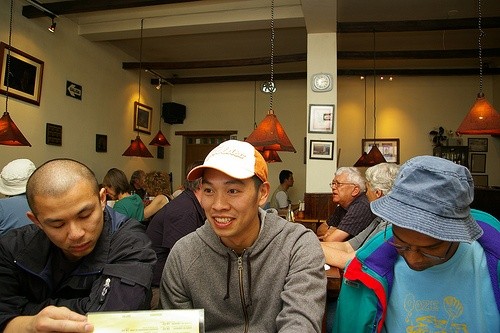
[294,214,329,234]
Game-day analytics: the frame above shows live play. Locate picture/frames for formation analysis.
[308,104,335,134]
[133,101,153,135]
[0,42,44,106]
[362,138,400,165]
[310,140,334,160]
[468,138,488,187]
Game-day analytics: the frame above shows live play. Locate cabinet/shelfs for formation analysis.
[433,146,469,168]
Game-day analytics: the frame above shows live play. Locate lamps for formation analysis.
[0,0,32,147]
[456,0,500,135]
[48,17,56,33]
[149,76,171,147]
[352,21,387,167]
[245,0,297,163]
[122,20,154,158]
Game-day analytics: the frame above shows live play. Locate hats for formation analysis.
[187,139,268,183]
[370,155,484,244]
[0,159,36,195]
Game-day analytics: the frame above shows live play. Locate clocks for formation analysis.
[312,73,333,93]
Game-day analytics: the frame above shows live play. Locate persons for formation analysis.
[160,140,327,333]
[0,159,37,234]
[270,170,303,219]
[0,158,158,333]
[317,167,378,242]
[143,171,174,221]
[102,168,145,224]
[320,162,402,271]
[172,182,186,198]
[128,170,149,201]
[332,155,500,333]
[145,161,207,288]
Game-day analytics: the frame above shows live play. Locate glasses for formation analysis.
[384,221,454,262]
[328,181,355,187]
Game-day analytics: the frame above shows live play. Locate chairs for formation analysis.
[145,172,173,200]
